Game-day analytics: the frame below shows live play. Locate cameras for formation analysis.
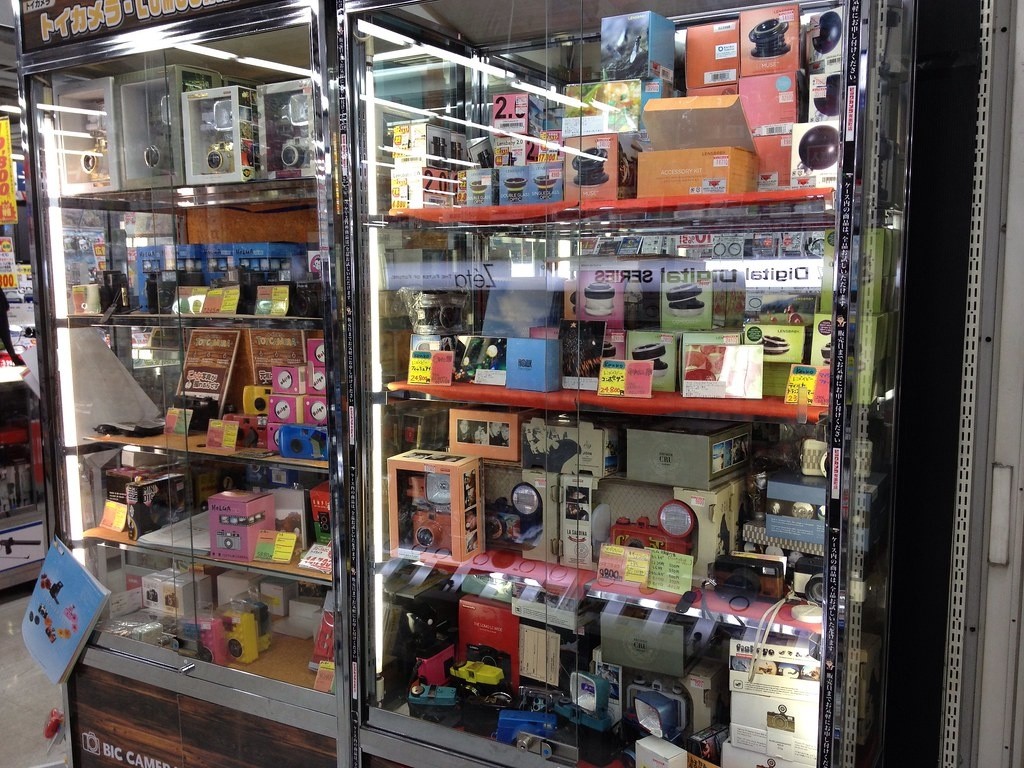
[82,145,111,186]
[223,414,268,450]
[243,385,273,416]
[175,395,219,432]
[207,99,252,174]
[144,94,173,176]
[485,483,543,544]
[408,643,687,768]
[210,267,266,314]
[216,531,240,550]
[611,499,695,557]
[184,599,272,666]
[279,424,329,462]
[146,270,205,315]
[412,472,453,551]
[794,556,825,608]
[281,93,319,169]
[98,271,131,316]
[713,556,784,602]
[291,282,330,319]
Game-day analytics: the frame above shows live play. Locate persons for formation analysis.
[0,288,29,368]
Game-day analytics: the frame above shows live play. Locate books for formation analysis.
[20,532,113,686]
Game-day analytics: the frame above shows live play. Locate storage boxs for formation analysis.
[52,62,313,193]
[268,338,327,452]
[310,479,333,549]
[141,568,325,643]
[504,227,885,399]
[207,489,276,562]
[391,0,842,210]
[457,594,883,768]
[106,464,186,542]
[387,404,892,592]
[135,241,308,315]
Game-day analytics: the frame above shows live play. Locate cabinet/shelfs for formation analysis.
[338,4,919,768]
[6,0,357,768]
